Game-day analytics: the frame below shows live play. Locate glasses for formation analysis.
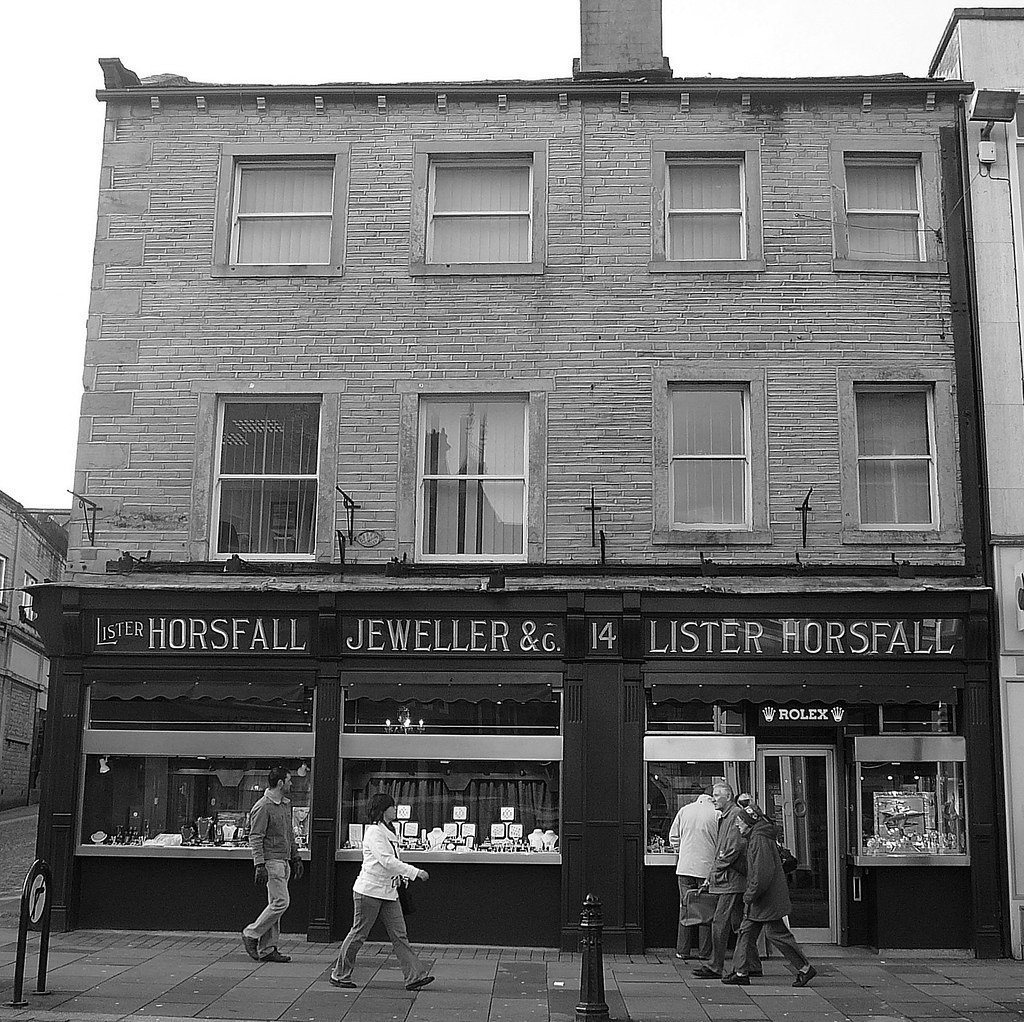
[389,805,397,810]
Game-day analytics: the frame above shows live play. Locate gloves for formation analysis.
[292,856,303,879]
[254,864,268,886]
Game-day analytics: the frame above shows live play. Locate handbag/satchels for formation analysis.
[679,886,720,927]
[729,855,748,877]
[775,843,797,873]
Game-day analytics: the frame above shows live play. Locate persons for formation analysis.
[329,792,435,991]
[669,782,818,987]
[241,768,304,963]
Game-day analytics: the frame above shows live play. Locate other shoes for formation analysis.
[329,977,357,988]
[698,952,707,959]
[693,966,722,978]
[792,965,817,987]
[242,933,259,960]
[260,947,291,962]
[676,949,690,959]
[724,972,736,979]
[406,977,435,989]
[721,974,750,985]
[745,969,763,976]
